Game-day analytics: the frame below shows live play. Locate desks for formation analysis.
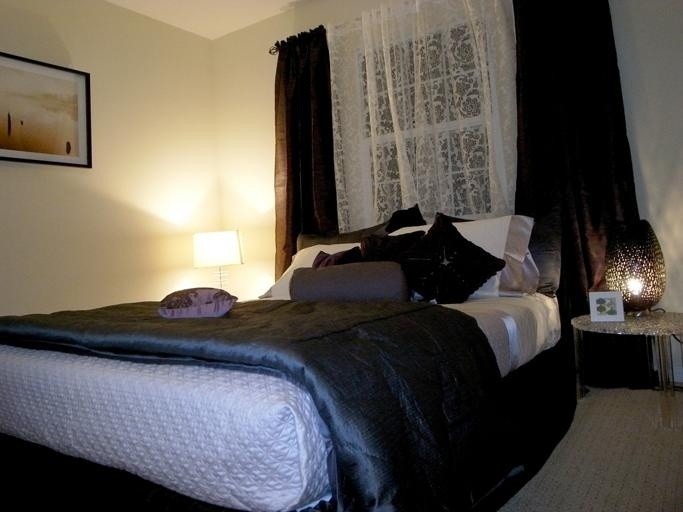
[571,312,683,426]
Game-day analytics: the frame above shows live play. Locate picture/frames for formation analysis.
[589,292,624,323]
[1,52,92,169]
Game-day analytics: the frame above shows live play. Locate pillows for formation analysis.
[312,245,362,268]
[401,213,507,305]
[296,204,427,251]
[158,287,238,317]
[289,261,408,305]
[361,230,426,262]
[436,208,563,299]
[258,244,362,301]
[388,214,541,299]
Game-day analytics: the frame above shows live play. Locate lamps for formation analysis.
[605,219,668,316]
[194,230,244,291]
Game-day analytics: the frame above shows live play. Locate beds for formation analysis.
[0,291,579,512]
